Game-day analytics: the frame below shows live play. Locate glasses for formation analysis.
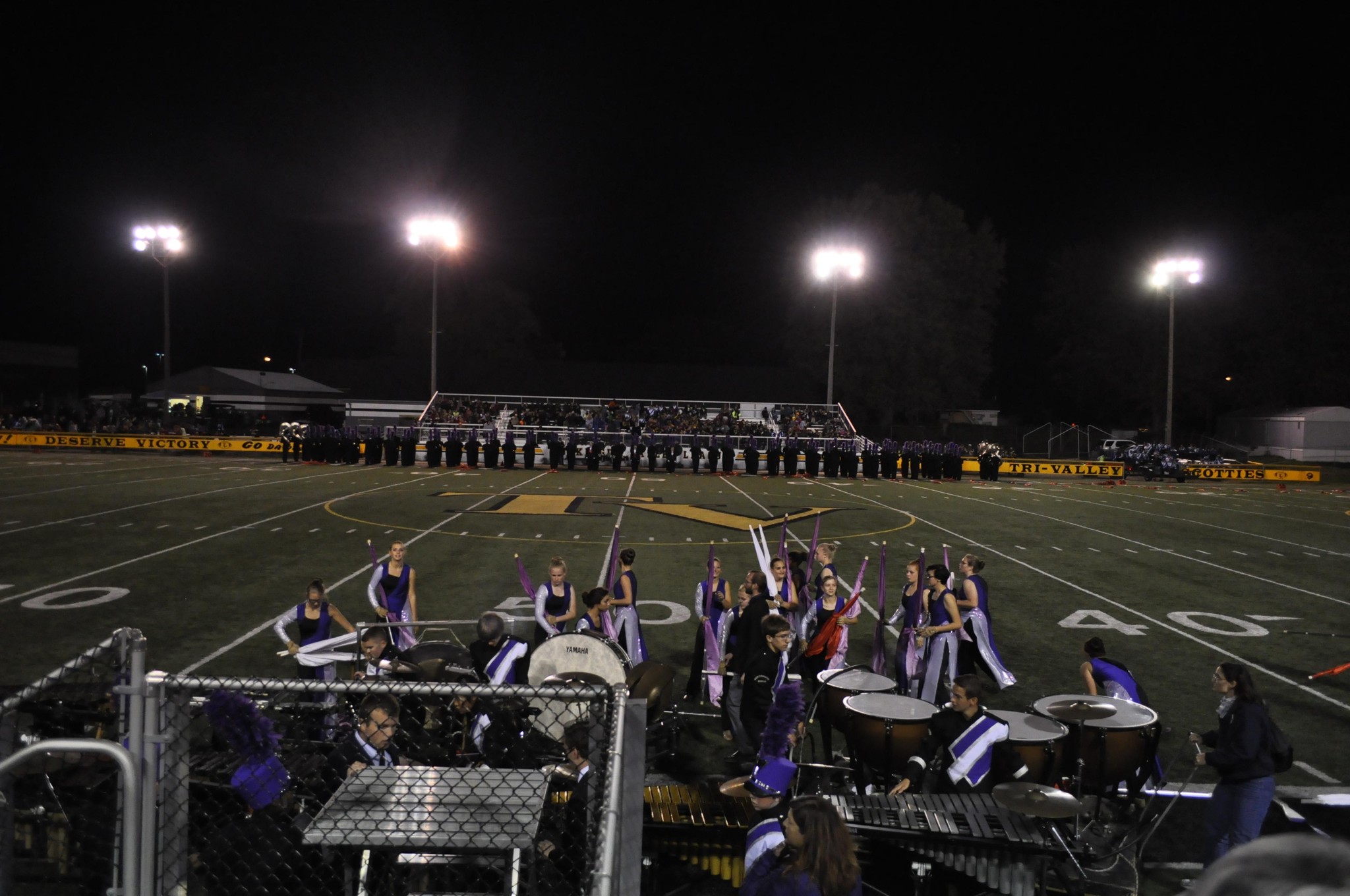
[961,561,970,565]
[925,576,935,579]
[1212,673,1228,683]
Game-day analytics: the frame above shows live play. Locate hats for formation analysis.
[203,688,288,809]
[743,680,806,798]
[295,425,962,459]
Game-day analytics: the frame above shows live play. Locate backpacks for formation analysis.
[1263,714,1294,774]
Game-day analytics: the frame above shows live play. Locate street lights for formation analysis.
[130,226,185,433]
[1154,256,1200,449]
[813,249,863,419]
[405,217,456,405]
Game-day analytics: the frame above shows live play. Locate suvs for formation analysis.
[1102,440,1143,458]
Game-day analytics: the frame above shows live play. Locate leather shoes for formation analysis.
[723,730,733,740]
[724,750,748,762]
[683,694,694,700]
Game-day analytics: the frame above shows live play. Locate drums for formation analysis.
[843,692,944,768]
[525,629,632,744]
[817,668,899,728]
[275,702,322,741]
[981,710,1069,779]
[1035,695,1158,775]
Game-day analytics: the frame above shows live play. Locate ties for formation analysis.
[378,747,386,767]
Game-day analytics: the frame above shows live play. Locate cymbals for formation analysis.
[796,763,857,772]
[1044,699,1118,719]
[628,659,675,721]
[719,775,761,797]
[993,782,1084,818]
[324,702,355,715]
[368,659,424,675]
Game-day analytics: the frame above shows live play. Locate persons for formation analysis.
[280,422,419,466]
[686,542,1016,750]
[737,796,861,896]
[530,548,650,673]
[354,626,401,681]
[536,718,654,887]
[1079,636,1168,789]
[0,400,281,438]
[1189,662,1277,874]
[419,393,853,448]
[367,541,419,675]
[314,692,400,896]
[887,674,1035,796]
[426,429,577,471]
[274,578,358,741]
[743,757,798,878]
[584,432,735,474]
[1095,442,1225,475]
[464,612,530,750]
[862,438,1015,482]
[743,436,859,479]
